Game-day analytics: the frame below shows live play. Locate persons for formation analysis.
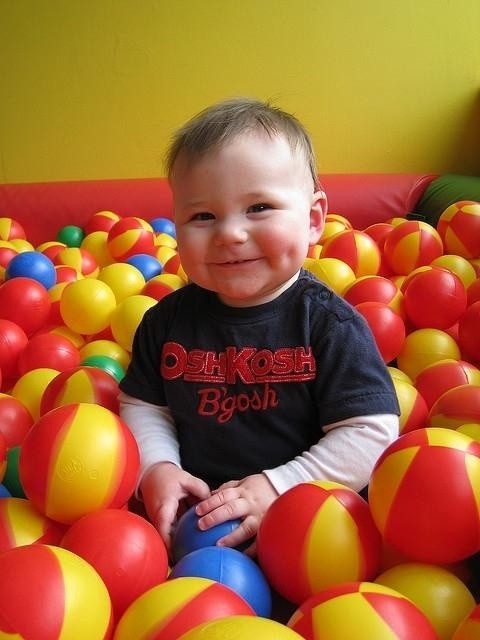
[118,102,399,561]
